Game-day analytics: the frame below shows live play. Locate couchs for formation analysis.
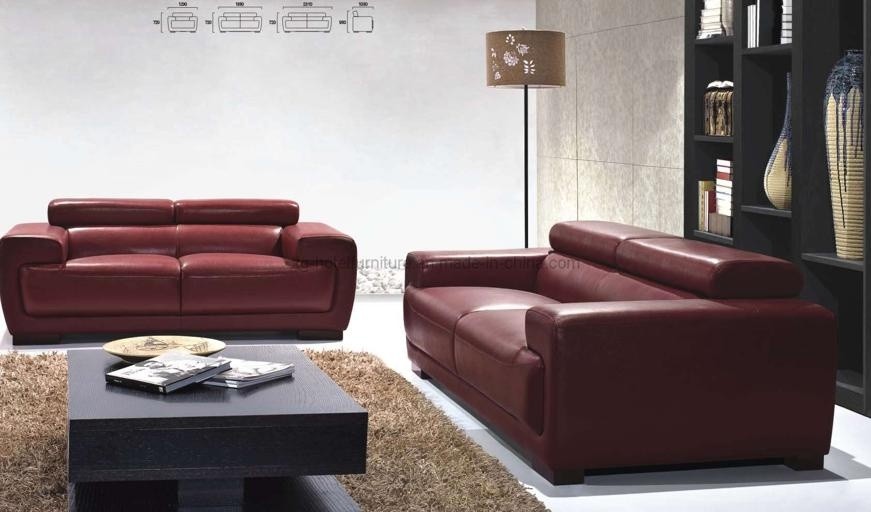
[403,218,839,489]
[0,195,358,346]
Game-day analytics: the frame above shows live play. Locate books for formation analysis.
[695,0,793,51]
[197,355,294,390]
[696,157,733,238]
[103,346,232,396]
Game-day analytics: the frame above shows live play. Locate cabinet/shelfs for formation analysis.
[681,0,871,418]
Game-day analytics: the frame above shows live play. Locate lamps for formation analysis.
[484,25,566,248]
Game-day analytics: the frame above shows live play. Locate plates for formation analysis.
[103,335,227,361]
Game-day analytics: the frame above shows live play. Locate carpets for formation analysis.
[0,349,551,511]
